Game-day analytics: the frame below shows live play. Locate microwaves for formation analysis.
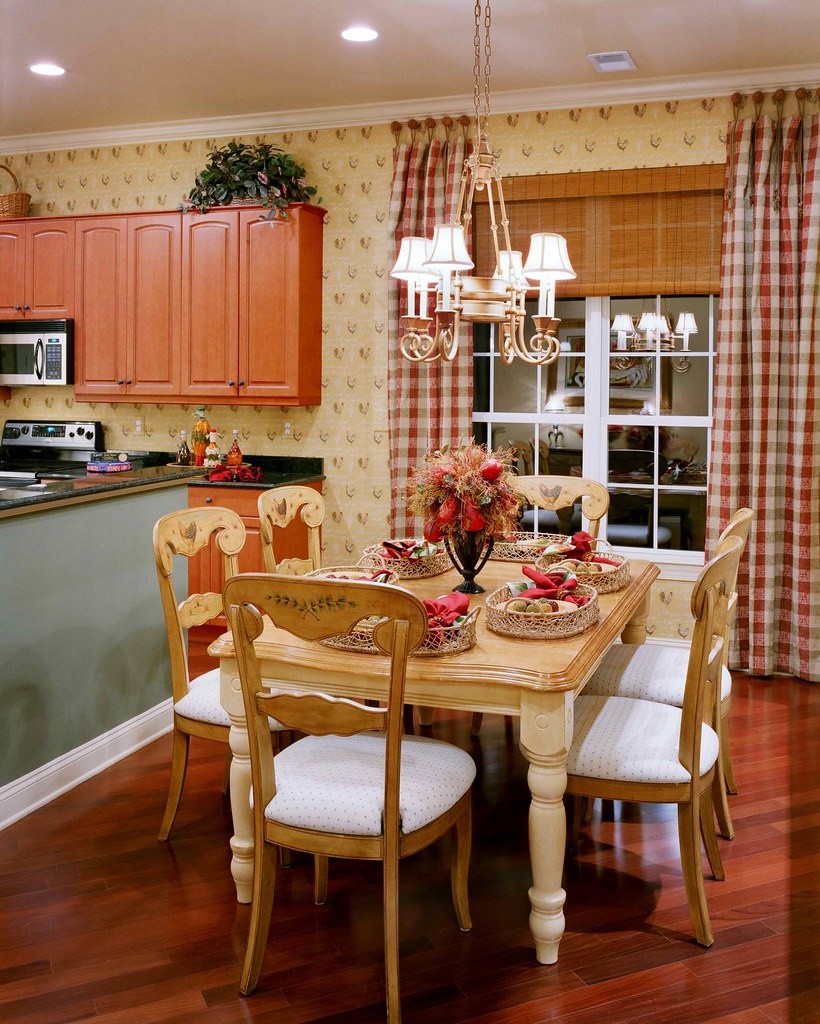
[0,318,75,387]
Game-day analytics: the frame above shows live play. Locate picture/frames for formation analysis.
[544,318,674,411]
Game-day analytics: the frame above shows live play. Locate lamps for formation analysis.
[544,397,564,448]
[610,293,699,374]
[389,0,578,366]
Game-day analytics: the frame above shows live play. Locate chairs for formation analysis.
[529,437,583,511]
[542,446,676,550]
[221,571,477,1024]
[504,474,611,551]
[507,439,580,535]
[255,485,417,736]
[152,505,334,868]
[561,534,746,947]
[579,508,753,839]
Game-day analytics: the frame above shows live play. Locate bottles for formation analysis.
[226,430,242,466]
[205,428,220,468]
[191,407,211,466]
[176,430,191,466]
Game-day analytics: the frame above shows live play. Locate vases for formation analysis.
[444,530,496,595]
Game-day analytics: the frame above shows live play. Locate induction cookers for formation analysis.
[0,419,104,486]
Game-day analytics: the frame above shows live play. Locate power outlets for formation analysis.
[133,415,146,436]
[281,417,296,440]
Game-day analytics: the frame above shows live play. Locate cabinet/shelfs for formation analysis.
[187,486,322,682]
[0,200,328,408]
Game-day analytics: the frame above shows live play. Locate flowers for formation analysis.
[403,432,523,542]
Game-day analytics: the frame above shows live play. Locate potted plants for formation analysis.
[175,141,317,219]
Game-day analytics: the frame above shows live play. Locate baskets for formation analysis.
[320,621,383,653]
[609,474,652,485]
[536,537,631,593]
[682,464,707,483]
[485,566,600,640]
[411,606,481,655]
[481,532,566,564]
[363,538,459,578]
[296,550,401,590]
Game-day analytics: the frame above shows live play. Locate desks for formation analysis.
[207,555,662,967]
[563,462,707,553]
